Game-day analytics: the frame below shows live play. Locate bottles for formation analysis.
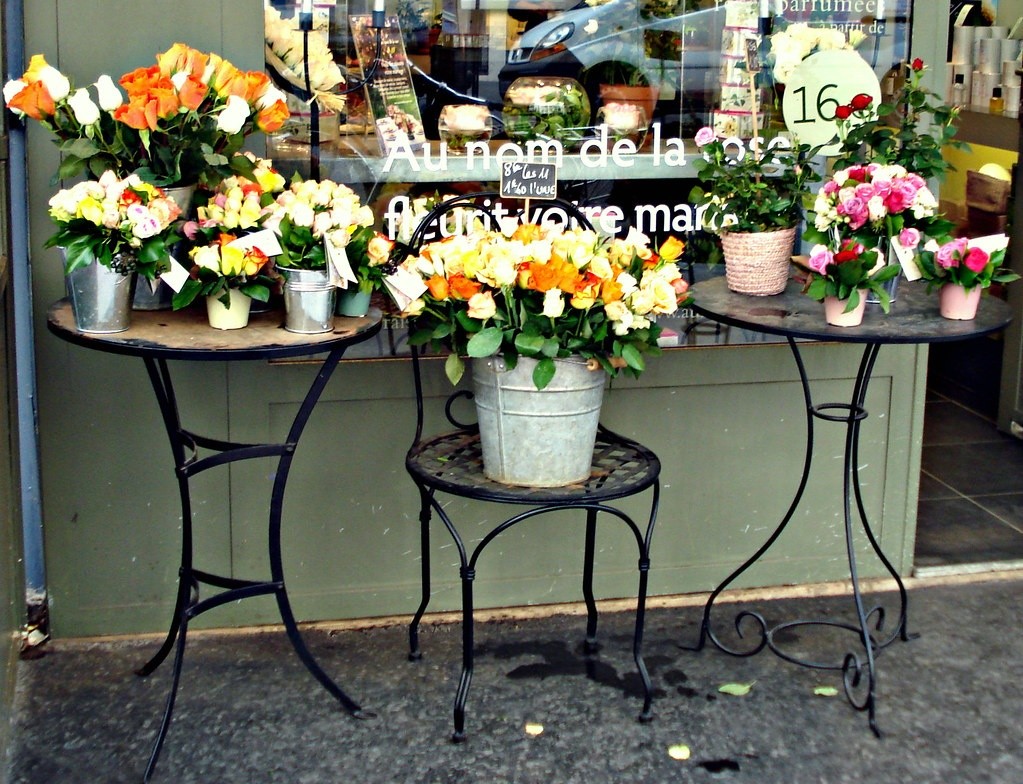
[952,74,967,109]
[990,87,1004,116]
[439,33,490,47]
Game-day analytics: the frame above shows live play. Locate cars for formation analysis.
[497,0,725,139]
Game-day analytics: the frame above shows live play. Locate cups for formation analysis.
[594,106,648,153]
[946,25,1021,112]
[438,104,493,155]
[504,78,591,154]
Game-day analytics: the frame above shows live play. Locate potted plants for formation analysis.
[393,0,432,75]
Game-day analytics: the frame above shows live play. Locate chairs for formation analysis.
[378,182,663,741]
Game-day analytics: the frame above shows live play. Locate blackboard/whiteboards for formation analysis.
[500,161,557,200]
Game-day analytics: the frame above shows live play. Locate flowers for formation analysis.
[575,8,691,91]
[903,225,1021,293]
[398,194,696,394]
[787,237,903,316]
[831,57,978,184]
[256,171,374,291]
[687,125,843,236]
[355,229,402,293]
[503,84,585,149]
[440,105,489,153]
[3,43,286,311]
[802,161,958,282]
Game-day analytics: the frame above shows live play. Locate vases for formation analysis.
[599,83,662,147]
[937,283,982,321]
[719,225,799,297]
[501,76,590,151]
[824,288,869,328]
[437,105,493,156]
[831,224,903,303]
[206,289,252,331]
[333,277,374,317]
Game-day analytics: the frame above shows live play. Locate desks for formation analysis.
[46,294,384,784]
[681,268,1014,742]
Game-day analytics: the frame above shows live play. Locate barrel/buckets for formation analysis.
[56,246,140,334]
[470,346,604,487]
[276,263,338,333]
[133,182,200,311]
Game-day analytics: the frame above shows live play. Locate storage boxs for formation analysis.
[966,169,1013,213]
[967,206,1006,237]
